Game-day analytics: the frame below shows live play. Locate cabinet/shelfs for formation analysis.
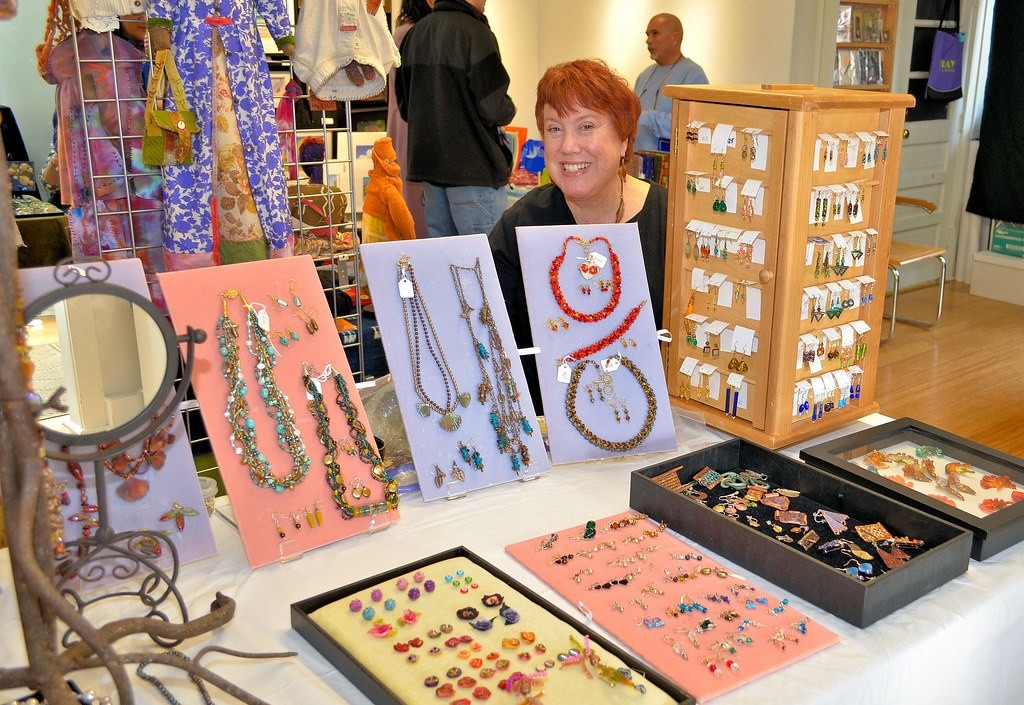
[661,81,917,447]
[832,0,899,93]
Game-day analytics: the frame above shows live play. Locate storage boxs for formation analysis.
[798,417,1023,562]
[627,435,975,631]
[290,545,696,705]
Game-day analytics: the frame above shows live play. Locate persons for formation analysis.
[293,0,401,102]
[488,59,665,414]
[386,0,517,240]
[632,13,710,184]
[146,0,295,272]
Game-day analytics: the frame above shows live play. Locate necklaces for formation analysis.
[545,233,658,452]
[395,250,533,477]
[12,275,173,580]
[216,287,397,520]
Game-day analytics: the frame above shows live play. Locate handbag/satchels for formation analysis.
[142,49,202,165]
[925,1,963,104]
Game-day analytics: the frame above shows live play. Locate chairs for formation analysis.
[879,196,947,344]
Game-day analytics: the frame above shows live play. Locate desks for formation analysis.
[0,414,1023,705]
